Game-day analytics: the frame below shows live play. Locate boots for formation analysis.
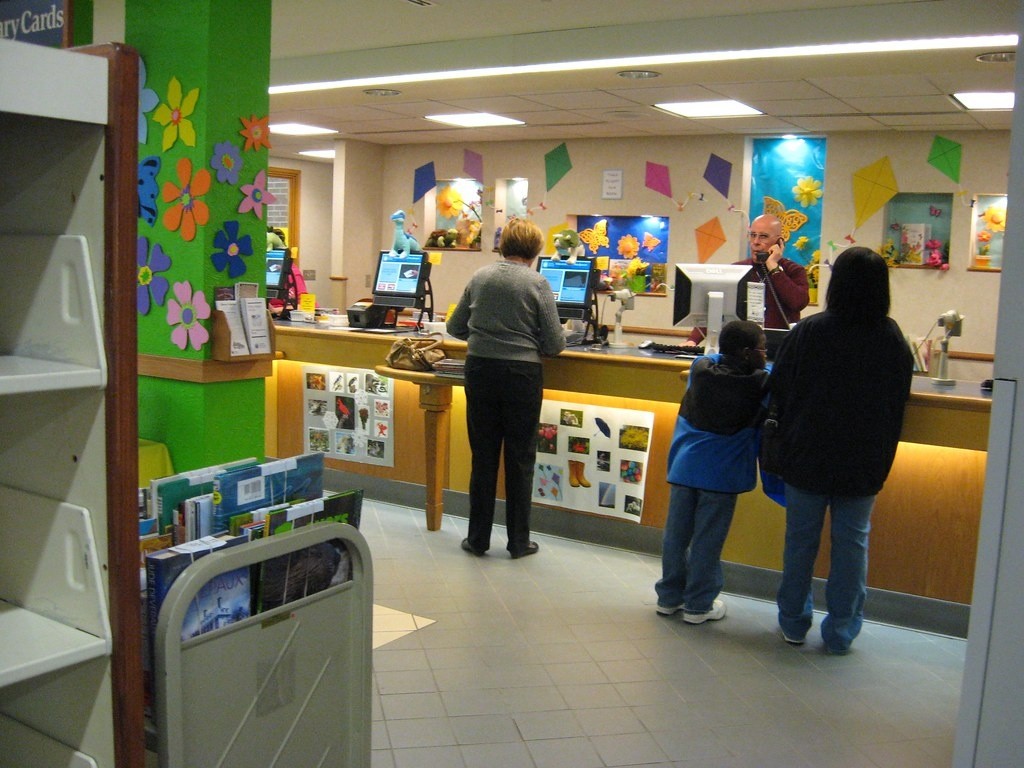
[576,461,591,488]
[569,460,580,487]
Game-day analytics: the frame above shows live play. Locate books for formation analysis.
[134,448,365,725]
[432,359,466,380]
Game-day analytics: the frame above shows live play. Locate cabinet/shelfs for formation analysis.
[0,36,146,768]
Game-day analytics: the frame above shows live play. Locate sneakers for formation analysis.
[656,603,685,615]
[683,600,726,624]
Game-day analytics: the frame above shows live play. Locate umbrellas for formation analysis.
[594,417,610,439]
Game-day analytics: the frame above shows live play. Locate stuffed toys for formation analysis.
[425,228,458,248]
[266,226,286,252]
[388,210,420,258]
[551,229,585,264]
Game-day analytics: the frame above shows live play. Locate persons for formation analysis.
[447,218,567,559]
[680,214,810,347]
[766,247,915,655]
[268,262,320,311]
[654,320,771,625]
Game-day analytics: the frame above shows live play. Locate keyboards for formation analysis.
[653,344,705,355]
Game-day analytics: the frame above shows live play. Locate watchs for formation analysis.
[768,265,784,276]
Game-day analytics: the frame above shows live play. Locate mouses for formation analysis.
[638,340,655,349]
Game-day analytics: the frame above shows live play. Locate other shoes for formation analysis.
[461,538,485,557]
[782,632,804,644]
[510,541,538,559]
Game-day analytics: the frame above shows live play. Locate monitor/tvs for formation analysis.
[536,256,596,309]
[266,247,292,290]
[372,250,429,298]
[672,263,754,360]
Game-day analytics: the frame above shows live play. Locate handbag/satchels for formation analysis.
[385,332,446,371]
[763,380,834,493]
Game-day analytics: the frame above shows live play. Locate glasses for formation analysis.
[745,347,768,357]
[747,232,783,240]
